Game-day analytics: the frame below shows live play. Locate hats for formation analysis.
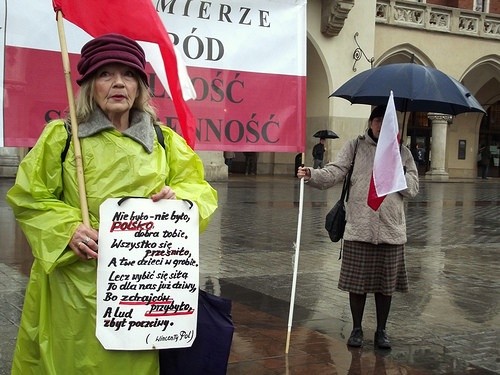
[75,34,149,88]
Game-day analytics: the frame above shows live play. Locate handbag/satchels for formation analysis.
[325,200,347,243]
[158,289,233,375]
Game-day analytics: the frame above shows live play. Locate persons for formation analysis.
[224,151,235,176]
[481,144,488,180]
[5,33,219,375]
[243,152,257,176]
[312,137,326,169]
[413,143,431,177]
[298,105,420,351]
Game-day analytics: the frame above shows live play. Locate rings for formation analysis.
[77,242,83,248]
[83,236,90,244]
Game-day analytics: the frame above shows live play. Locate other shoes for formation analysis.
[228,172,232,175]
[347,330,363,346]
[252,171,255,175]
[374,332,392,349]
[245,172,248,176]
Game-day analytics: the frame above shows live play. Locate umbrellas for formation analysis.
[326,52,488,177]
[313,129,339,146]
[158,289,234,375]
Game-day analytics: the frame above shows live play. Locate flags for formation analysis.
[51,0,197,150]
[367,88,408,211]
